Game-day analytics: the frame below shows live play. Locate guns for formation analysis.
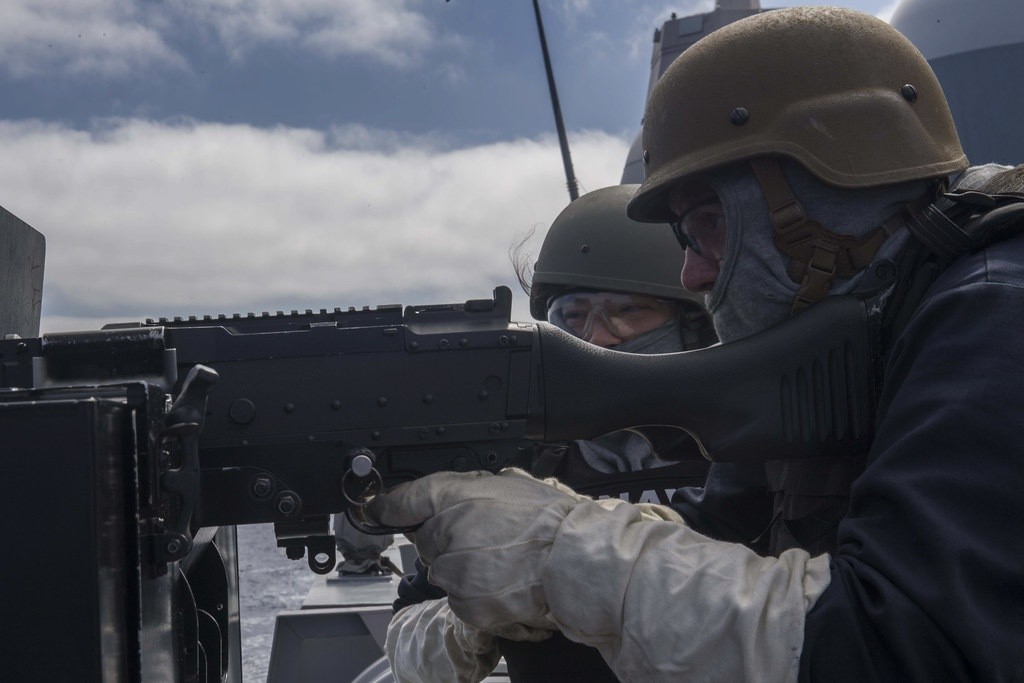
[0,282,892,583]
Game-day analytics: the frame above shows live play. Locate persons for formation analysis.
[361,11,1024,682]
[384,183,774,683]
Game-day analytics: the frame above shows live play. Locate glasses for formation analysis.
[546,287,690,342]
[672,197,726,261]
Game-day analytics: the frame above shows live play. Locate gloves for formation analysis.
[385,594,553,683]
[363,464,832,682]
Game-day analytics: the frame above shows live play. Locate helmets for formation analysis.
[529,183,714,329]
[625,8,971,222]
